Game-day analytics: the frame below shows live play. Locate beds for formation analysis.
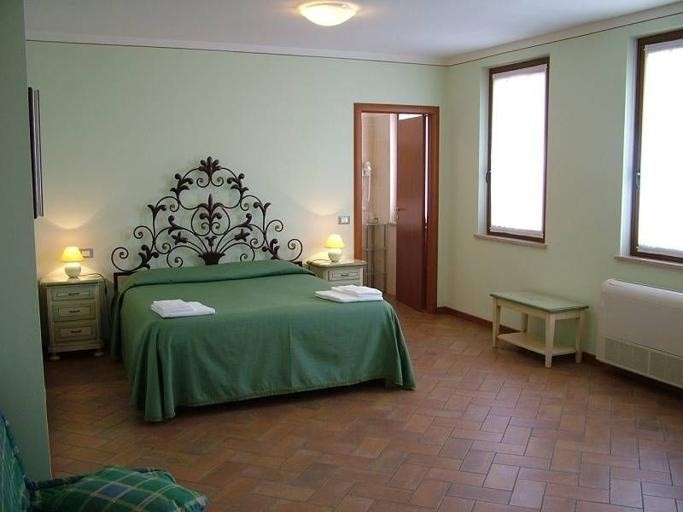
[108,152,407,422]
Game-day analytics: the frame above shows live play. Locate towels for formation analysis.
[148,295,216,319]
[313,282,384,303]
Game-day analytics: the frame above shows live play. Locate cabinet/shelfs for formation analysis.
[362,223,386,294]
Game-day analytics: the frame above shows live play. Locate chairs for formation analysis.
[0,408,213,512]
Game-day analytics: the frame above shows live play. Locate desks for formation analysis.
[487,285,591,370]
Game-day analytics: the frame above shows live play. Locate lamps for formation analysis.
[296,1,358,28]
[59,246,84,279]
[324,233,345,263]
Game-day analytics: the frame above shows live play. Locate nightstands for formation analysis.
[37,274,105,362]
[304,258,367,286]
